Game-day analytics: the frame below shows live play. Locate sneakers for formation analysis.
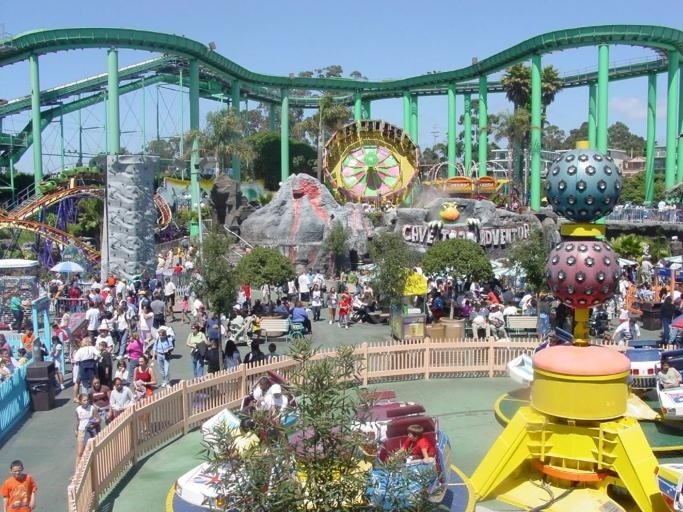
[329,319,349,330]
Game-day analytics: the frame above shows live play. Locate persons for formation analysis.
[1,222,683,512]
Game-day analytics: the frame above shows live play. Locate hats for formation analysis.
[97,325,112,333]
[137,291,145,295]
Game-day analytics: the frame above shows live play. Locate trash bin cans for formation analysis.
[25,361,55,410]
[425,325,444,340]
[641,302,663,330]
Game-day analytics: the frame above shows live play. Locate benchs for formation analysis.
[655,350,682,386]
[289,318,304,338]
[260,319,289,343]
[466,315,538,336]
[360,389,443,483]
